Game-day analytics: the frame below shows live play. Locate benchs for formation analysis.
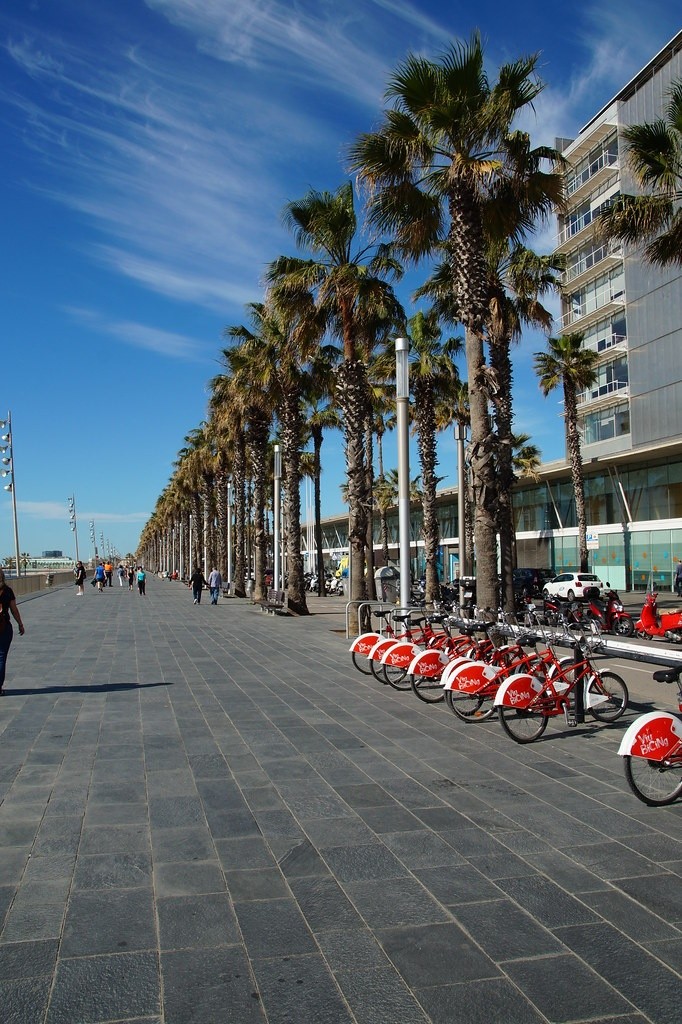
[218,582,230,598]
[255,589,285,615]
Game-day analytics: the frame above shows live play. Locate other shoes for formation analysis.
[76,592,84,596]
[194,599,197,603]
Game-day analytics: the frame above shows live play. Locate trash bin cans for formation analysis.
[374,566,400,602]
[342,568,349,599]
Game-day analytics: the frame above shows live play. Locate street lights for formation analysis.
[68,492,79,562]
[180,523,183,581]
[100,530,105,559]
[89,518,96,568]
[273,445,282,592]
[227,483,233,595]
[190,515,193,580]
[0,410,20,577]
[396,338,410,643]
[160,534,169,571]
[173,529,175,574]
[106,537,115,562]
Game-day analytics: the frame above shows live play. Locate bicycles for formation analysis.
[617,666,682,807]
[348,598,629,744]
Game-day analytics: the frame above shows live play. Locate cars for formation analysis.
[441,568,557,606]
[542,572,604,603]
[245,567,273,586]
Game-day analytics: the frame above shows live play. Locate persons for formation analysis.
[127,566,136,591]
[674,559,682,597]
[117,565,125,587]
[208,567,222,605]
[189,568,209,605]
[102,561,114,587]
[136,566,146,596]
[0,570,25,695]
[95,563,107,592]
[75,561,85,595]
[162,570,177,582]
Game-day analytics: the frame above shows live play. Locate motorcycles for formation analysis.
[278,571,344,596]
[411,577,635,637]
[633,593,682,644]
